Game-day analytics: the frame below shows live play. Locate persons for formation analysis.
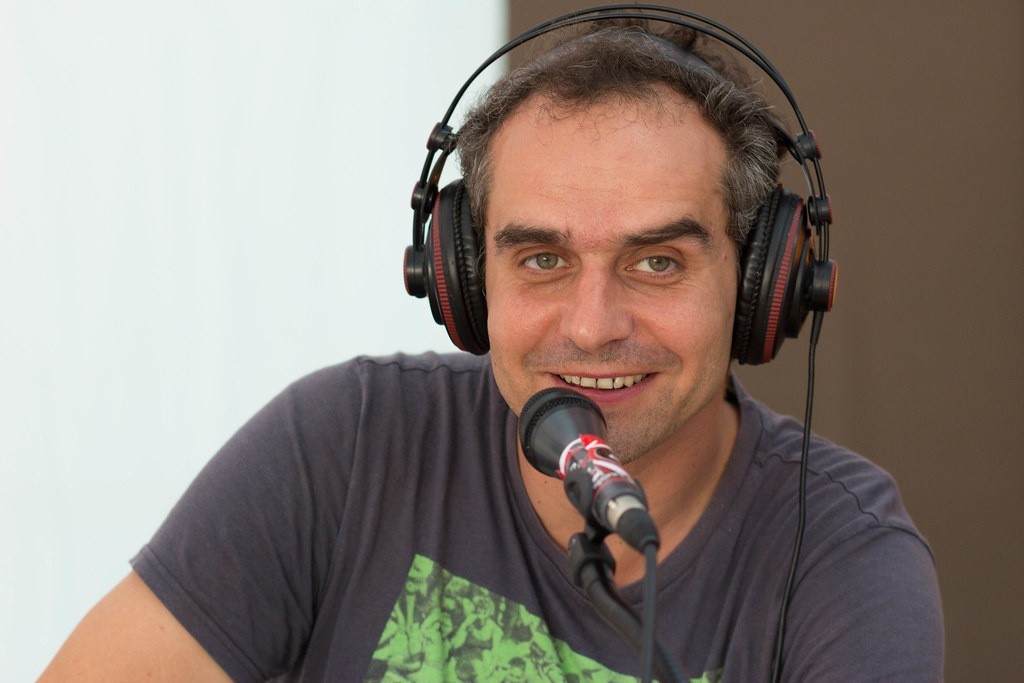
[37,17,944,683]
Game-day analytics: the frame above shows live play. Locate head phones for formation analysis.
[403,3,836,366]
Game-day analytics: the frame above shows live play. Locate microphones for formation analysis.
[519,387,660,553]
